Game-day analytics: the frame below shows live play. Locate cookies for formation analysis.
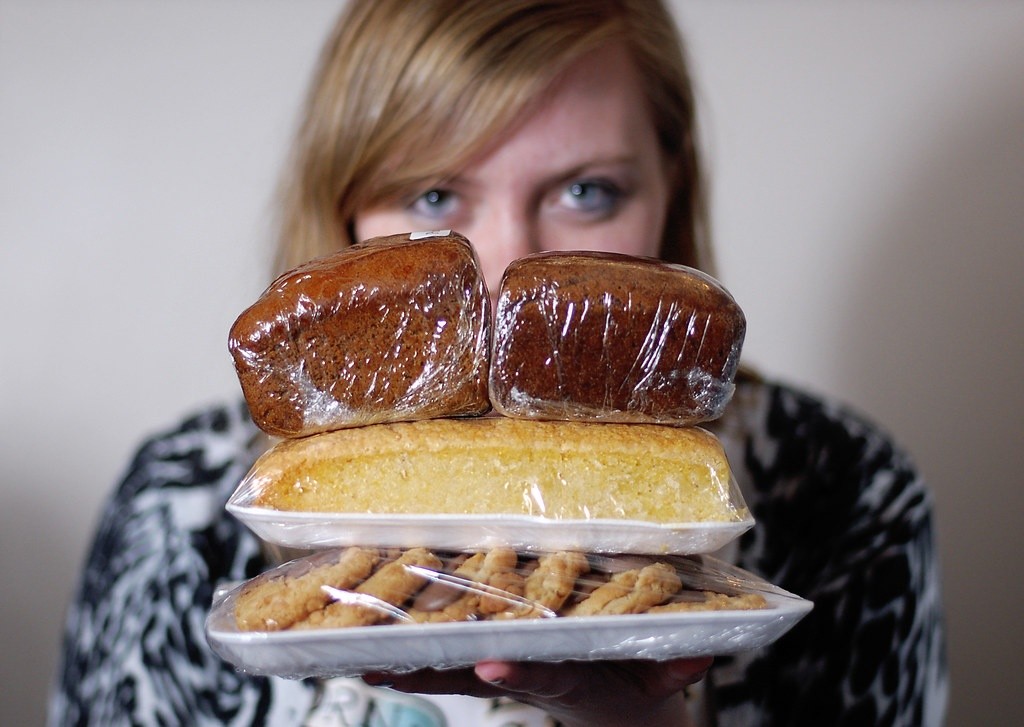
[232,544,768,631]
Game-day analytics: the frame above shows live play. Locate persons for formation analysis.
[52,0,949,727]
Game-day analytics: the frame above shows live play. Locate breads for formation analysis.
[226,230,746,524]
